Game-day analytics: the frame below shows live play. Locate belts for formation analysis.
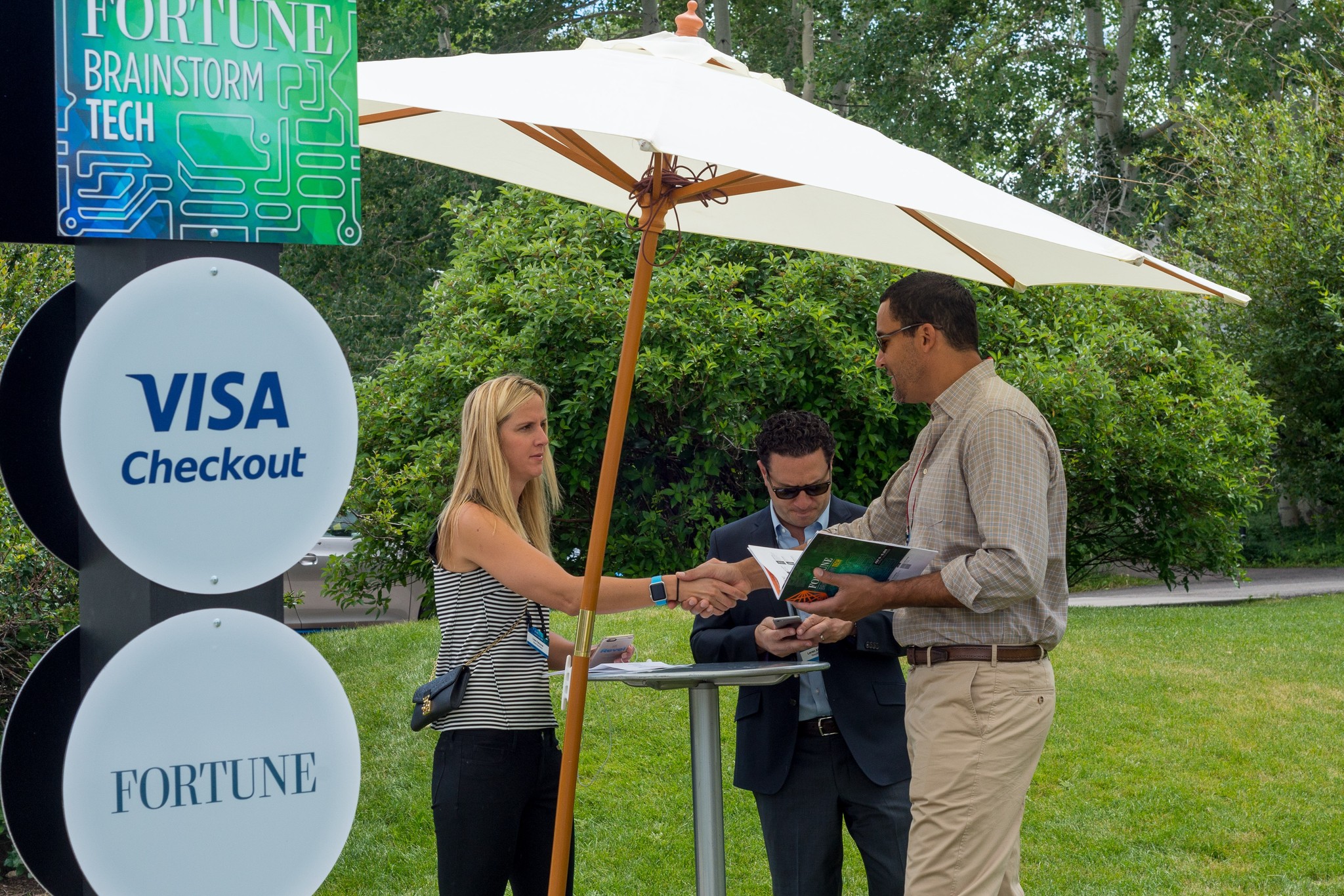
[796,714,840,736]
[907,645,1049,666]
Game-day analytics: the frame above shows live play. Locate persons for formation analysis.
[408,374,748,896]
[674,270,1068,896]
[690,412,911,896]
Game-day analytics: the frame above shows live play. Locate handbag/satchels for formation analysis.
[410,666,471,730]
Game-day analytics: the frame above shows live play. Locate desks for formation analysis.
[587,660,831,896]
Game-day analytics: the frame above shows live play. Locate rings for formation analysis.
[820,633,825,641]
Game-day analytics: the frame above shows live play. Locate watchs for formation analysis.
[649,575,668,606]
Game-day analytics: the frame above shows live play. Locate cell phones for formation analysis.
[773,616,802,641]
[589,634,635,667]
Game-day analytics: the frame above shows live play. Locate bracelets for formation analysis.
[676,578,679,601]
[852,622,857,637]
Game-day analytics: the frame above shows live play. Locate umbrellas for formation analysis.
[356,0,1254,896]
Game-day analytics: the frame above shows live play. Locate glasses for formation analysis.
[765,463,832,500]
[874,323,946,353]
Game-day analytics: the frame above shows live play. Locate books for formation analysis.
[748,531,938,612]
